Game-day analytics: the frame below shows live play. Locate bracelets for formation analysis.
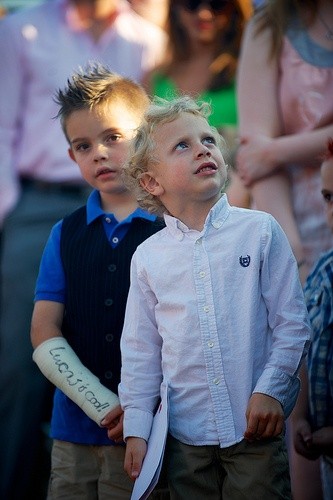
[296,257,307,267]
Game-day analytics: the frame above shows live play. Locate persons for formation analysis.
[120,96,310,500]
[130,0,250,208]
[1,0,168,500]
[28,59,165,500]
[234,0,333,500]
[291,138,333,500]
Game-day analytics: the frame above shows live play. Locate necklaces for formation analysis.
[317,10,333,41]
